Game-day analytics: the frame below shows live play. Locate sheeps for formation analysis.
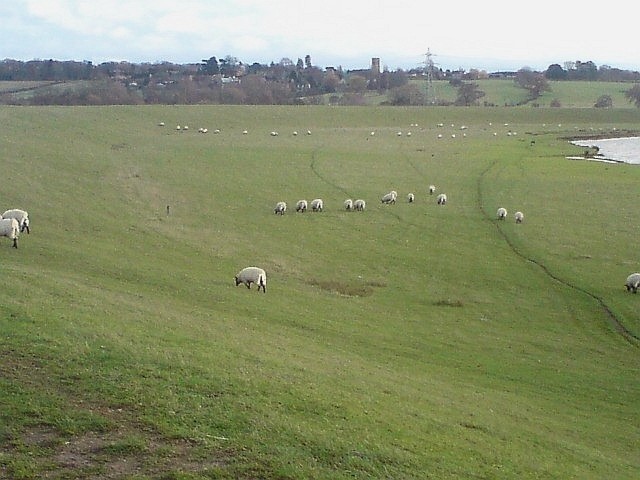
[234,266,267,294]
[407,192,415,203]
[380,191,398,205]
[353,199,366,211]
[429,184,436,195]
[437,193,447,205]
[295,199,307,213]
[0,215,20,249]
[273,201,287,216]
[311,198,323,212]
[344,198,353,210]
[624,273,640,294]
[514,211,524,224]
[496,207,507,222]
[3,208,30,234]
